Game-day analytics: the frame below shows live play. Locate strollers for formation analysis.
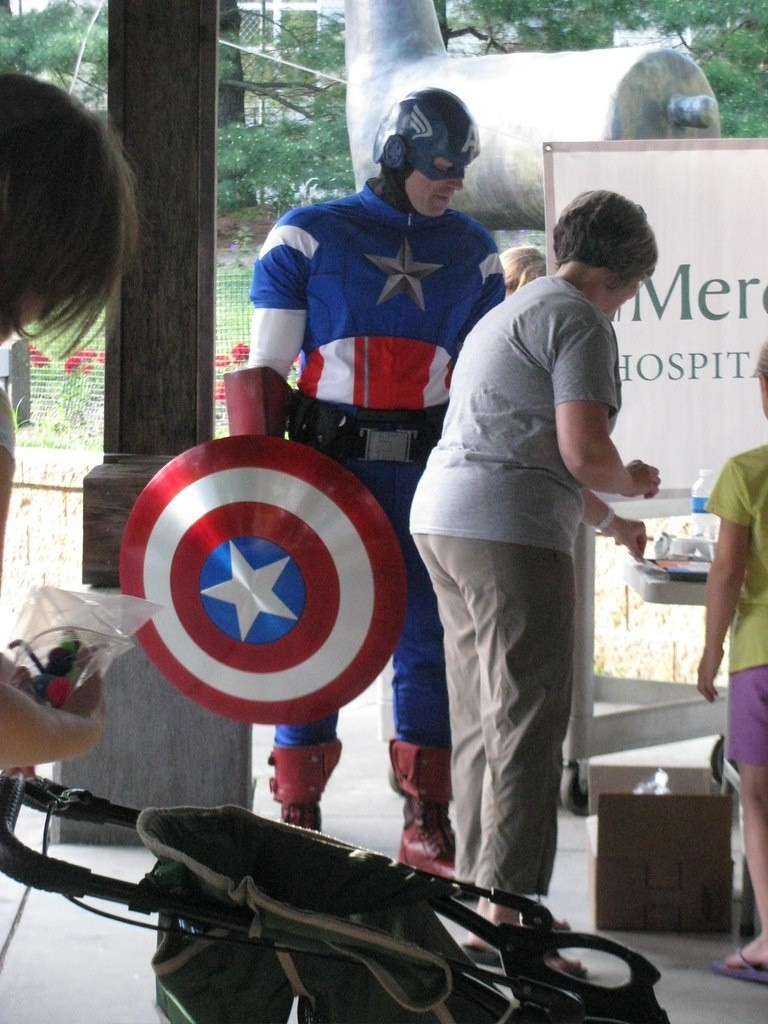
[0,769,672,1022]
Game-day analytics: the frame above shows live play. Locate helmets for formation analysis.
[373,85,481,181]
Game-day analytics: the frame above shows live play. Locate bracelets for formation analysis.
[593,507,616,532]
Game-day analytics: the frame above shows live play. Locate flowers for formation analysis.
[215,344,303,425]
[28,344,106,432]
[230,244,244,269]
[497,230,532,247]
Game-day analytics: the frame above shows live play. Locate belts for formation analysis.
[289,390,450,466]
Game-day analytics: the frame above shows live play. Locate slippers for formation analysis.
[713,946,766,985]
[460,941,588,979]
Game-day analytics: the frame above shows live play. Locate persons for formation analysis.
[223,84,508,878]
[401,191,661,969]
[494,244,553,293]
[696,339,768,987]
[0,74,140,792]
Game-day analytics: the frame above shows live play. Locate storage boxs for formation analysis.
[586,764,734,934]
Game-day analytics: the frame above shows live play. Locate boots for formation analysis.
[269,740,340,832]
[388,739,456,878]
